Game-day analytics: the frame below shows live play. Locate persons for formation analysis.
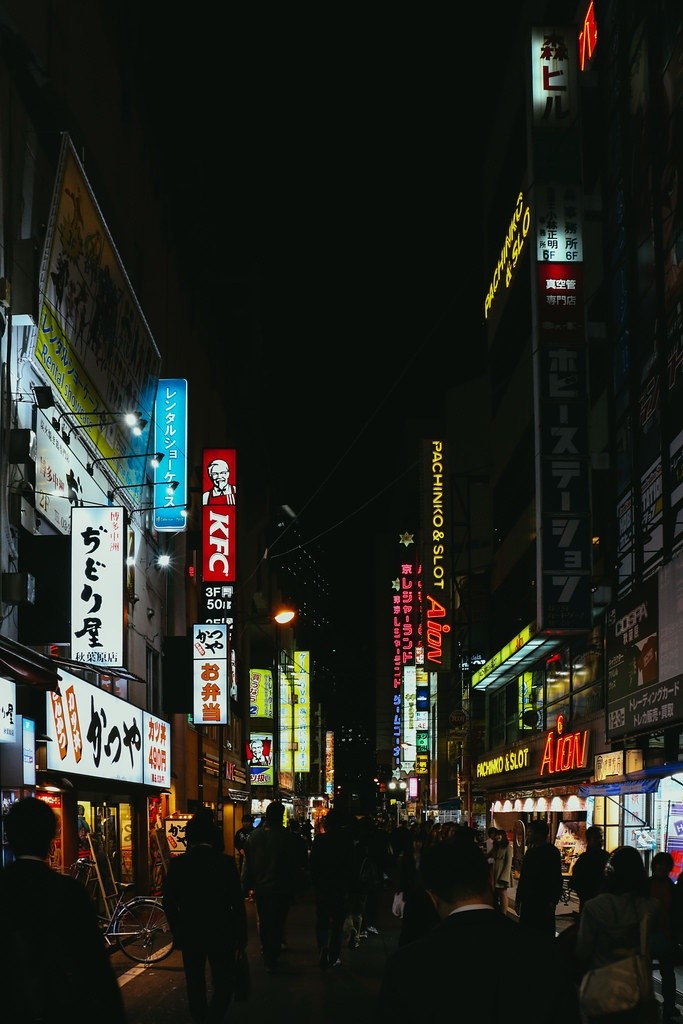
[567,826,608,915]
[644,852,681,1021]
[379,839,585,1024]
[514,820,563,987]
[0,799,126,1024]
[580,845,657,1024]
[163,813,248,1024]
[234,803,512,968]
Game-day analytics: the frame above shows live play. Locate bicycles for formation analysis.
[72,857,176,969]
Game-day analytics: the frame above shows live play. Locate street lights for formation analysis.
[401,743,431,821]
[217,607,297,852]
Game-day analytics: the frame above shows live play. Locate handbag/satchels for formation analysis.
[575,913,650,1016]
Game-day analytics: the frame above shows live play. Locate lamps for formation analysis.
[34,385,53,409]
[589,520,682,593]
[53,409,192,523]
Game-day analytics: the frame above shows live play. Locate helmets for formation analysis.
[586,826,603,841]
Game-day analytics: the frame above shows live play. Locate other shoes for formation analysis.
[346,926,358,951]
[317,947,342,969]
[360,926,379,939]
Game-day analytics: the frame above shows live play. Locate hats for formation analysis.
[242,815,255,823]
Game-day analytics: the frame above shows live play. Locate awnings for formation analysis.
[578,763,683,826]
